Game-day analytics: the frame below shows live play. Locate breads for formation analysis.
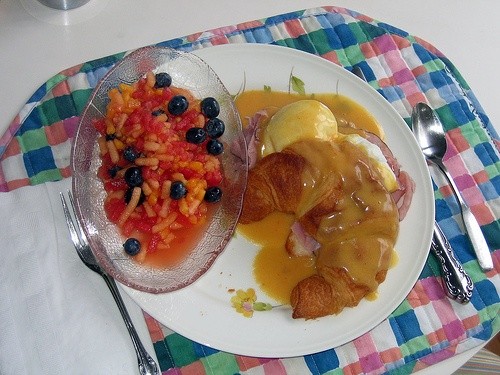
[237,149,305,224]
[289,268,364,321]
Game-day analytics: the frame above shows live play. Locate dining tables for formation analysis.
[0,7,500,375]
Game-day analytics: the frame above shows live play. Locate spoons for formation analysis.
[411,101,493,273]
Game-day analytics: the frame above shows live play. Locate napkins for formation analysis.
[0,170,163,374]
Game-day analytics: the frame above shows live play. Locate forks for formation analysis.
[59,190,161,375]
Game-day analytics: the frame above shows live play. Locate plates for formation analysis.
[71,46,248,295]
[116,44,436,359]
